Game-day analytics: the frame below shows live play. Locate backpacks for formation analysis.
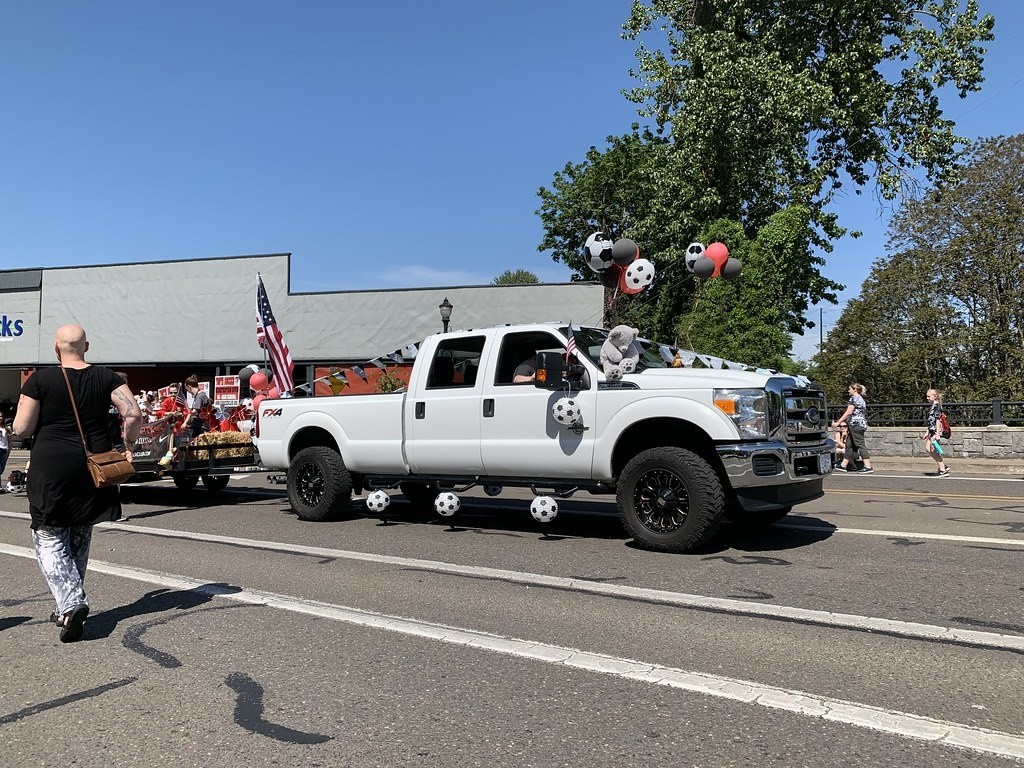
[935,404,951,439]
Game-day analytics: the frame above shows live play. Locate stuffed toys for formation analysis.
[599,325,638,381]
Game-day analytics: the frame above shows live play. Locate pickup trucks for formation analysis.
[252,321,838,556]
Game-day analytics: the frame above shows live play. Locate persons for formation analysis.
[513,354,544,383]
[133,383,191,448]
[923,389,950,478]
[0,412,12,494]
[108,372,129,522]
[180,374,211,438]
[832,383,873,474]
[13,325,143,643]
[835,421,859,471]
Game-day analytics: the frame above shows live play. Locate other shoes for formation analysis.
[0,486,5,493]
[116,514,129,522]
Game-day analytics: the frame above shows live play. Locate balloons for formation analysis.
[240,364,280,411]
[434,492,460,516]
[530,495,558,522]
[366,490,390,512]
[552,398,580,425]
[686,242,743,280]
[484,486,501,496]
[585,232,655,294]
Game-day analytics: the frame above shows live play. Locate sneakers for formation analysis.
[857,466,873,474]
[931,465,950,478]
[834,464,847,472]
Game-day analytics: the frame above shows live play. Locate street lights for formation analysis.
[438,296,454,334]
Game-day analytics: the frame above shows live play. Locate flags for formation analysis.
[255,277,295,393]
[567,323,575,356]
[176,389,186,406]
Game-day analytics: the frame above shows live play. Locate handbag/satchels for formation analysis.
[87,447,136,488]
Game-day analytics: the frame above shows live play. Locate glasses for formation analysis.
[839,425,848,428]
[169,391,178,394]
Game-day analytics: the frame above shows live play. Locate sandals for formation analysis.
[50,611,88,626]
[59,603,89,643]
